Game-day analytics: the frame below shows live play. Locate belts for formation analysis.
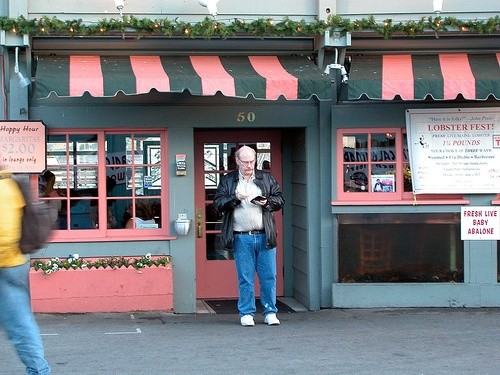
[233,229,265,235]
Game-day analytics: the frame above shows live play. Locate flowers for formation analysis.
[34,253,173,275]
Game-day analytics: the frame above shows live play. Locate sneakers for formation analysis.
[240,315,255,326]
[264,312,280,325]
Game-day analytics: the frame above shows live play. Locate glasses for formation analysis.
[238,156,255,165]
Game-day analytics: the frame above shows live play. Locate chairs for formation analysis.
[352,228,394,276]
[396,229,428,274]
[138,224,158,228]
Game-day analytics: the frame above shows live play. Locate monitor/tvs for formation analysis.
[372,174,395,192]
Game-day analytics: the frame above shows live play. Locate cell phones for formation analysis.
[250,196,266,205]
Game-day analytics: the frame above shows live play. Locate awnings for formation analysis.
[348,53,500,100]
[32,55,333,102]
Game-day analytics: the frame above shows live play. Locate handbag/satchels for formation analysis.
[0,169,61,255]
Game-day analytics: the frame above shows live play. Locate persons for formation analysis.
[213,147,284,326]
[0,161,51,375]
[124,195,155,228]
[40,172,60,229]
[90,176,115,229]
[374,179,384,192]
